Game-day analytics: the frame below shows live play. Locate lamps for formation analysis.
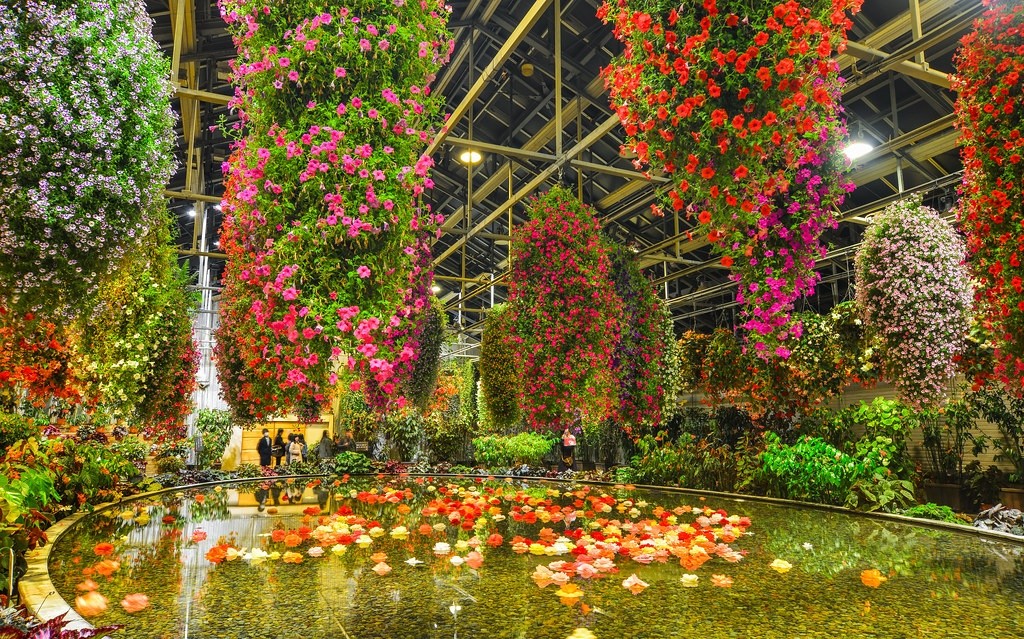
[522,63,534,77]
[938,193,955,212]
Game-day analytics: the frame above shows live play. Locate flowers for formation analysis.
[0,0,1024,639]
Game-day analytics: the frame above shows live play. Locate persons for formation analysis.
[562,429,574,458]
[254,481,328,510]
[258,428,357,467]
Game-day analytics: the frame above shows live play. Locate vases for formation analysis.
[924,482,965,513]
[574,460,583,471]
[999,487,1024,511]
[595,461,606,471]
[551,464,559,472]
